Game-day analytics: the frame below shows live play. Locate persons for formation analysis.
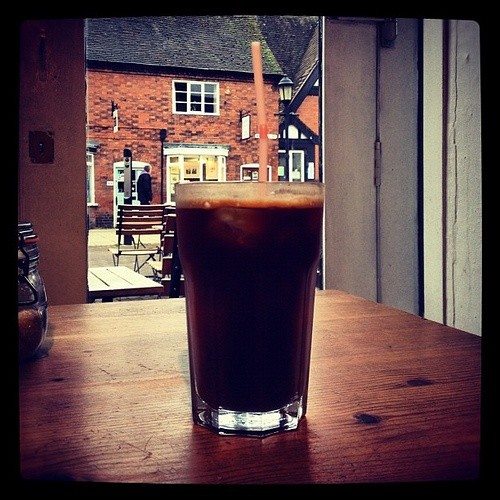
[137,166,153,205]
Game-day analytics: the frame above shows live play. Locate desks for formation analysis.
[21,288,483,486]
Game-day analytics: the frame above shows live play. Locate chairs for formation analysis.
[107,202,184,283]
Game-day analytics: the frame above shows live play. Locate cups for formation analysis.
[174,181,324,437]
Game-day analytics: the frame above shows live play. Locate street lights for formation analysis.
[277,72,294,181]
